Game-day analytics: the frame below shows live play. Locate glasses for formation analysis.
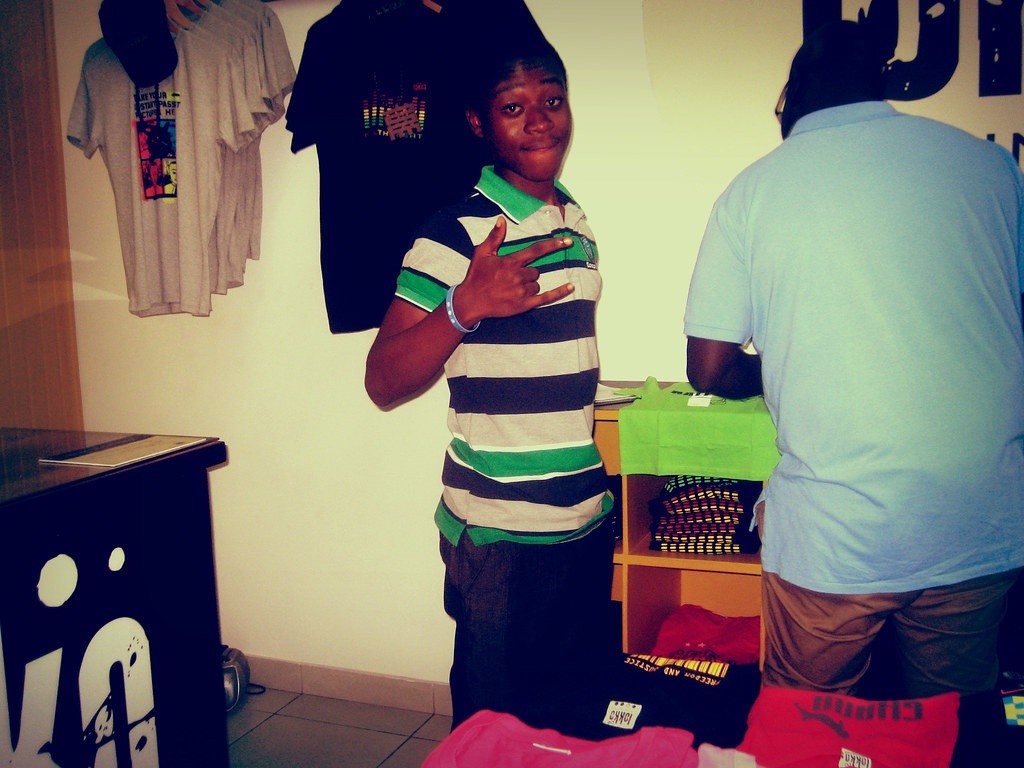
[775,82,791,122]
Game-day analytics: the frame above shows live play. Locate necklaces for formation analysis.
[134,83,172,157]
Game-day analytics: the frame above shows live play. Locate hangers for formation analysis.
[305,0,521,63]
[84,0,274,65]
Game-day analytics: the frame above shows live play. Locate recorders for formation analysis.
[221,647,251,713]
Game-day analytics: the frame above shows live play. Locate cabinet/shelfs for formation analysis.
[595,376,766,672]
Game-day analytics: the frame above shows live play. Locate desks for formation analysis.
[1,428,234,768]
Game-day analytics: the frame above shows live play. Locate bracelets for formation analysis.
[445,283,482,334]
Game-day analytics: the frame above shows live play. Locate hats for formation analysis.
[100,0,180,86]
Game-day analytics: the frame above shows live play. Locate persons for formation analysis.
[364,38,619,741]
[683,20,1023,767]
